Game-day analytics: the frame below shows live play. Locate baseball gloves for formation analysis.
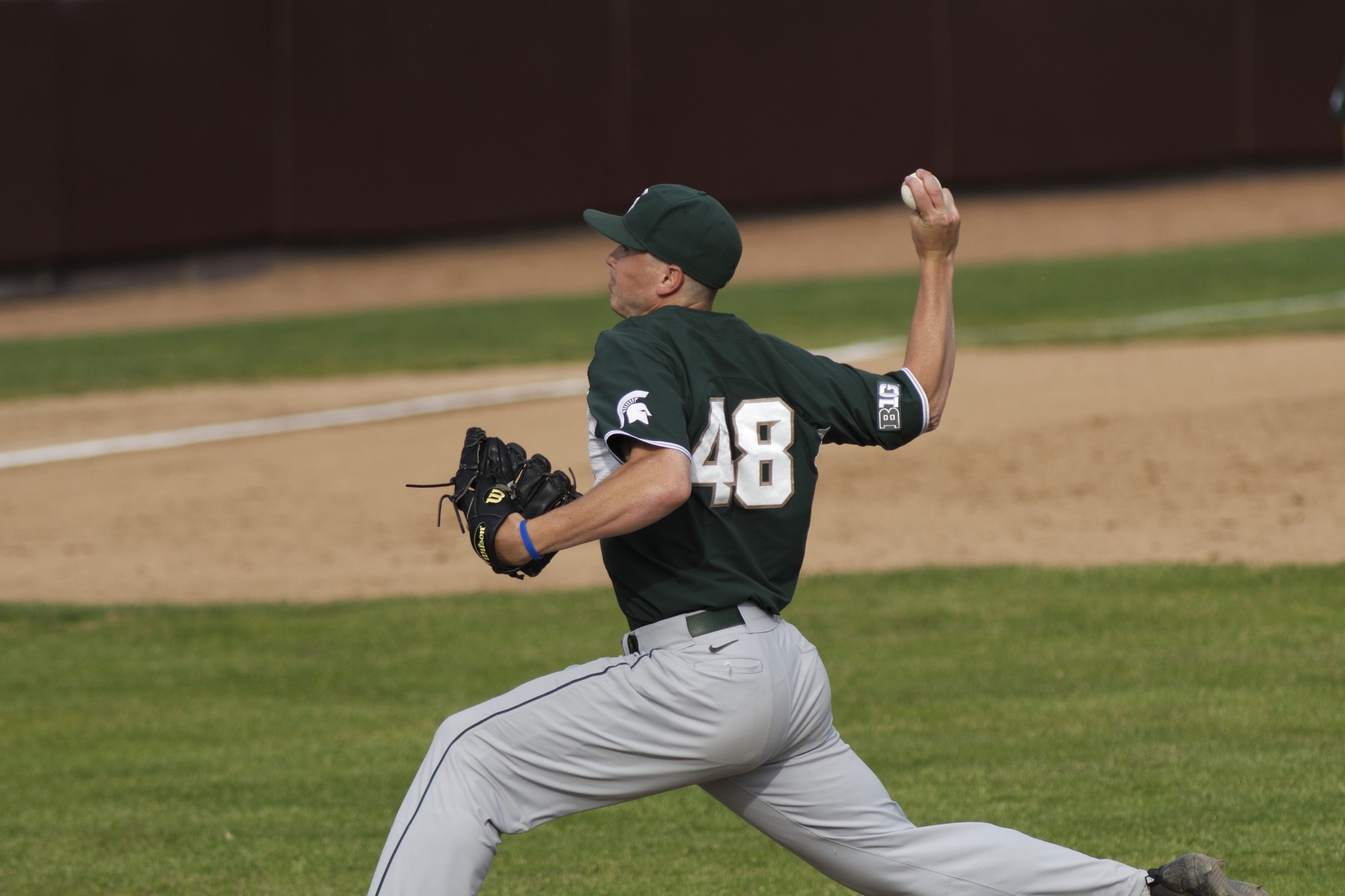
[449,427,585,577]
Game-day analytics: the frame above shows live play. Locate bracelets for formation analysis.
[520,519,544,560]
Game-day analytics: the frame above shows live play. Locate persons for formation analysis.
[367,169,1267,896]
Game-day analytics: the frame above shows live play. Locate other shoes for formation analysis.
[1146,852,1265,896]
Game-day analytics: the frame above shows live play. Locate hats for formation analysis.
[584,184,742,288]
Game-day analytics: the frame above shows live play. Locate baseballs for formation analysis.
[900,172,942,212]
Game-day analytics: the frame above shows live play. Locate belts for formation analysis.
[621,604,746,654]
